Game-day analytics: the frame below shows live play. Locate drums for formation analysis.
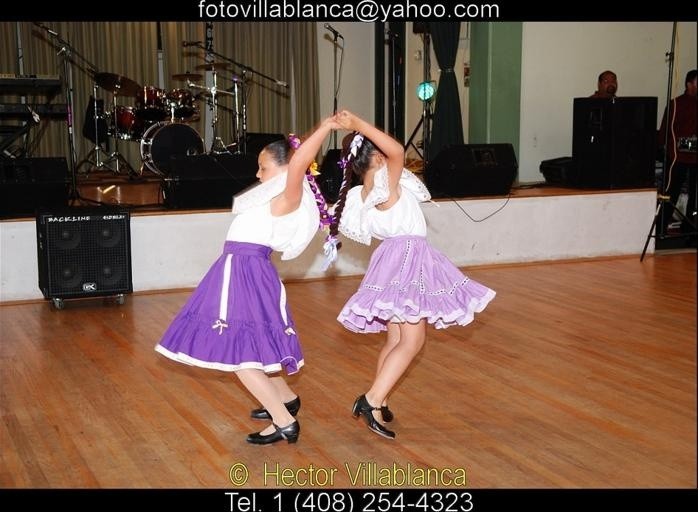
[168,89,194,117]
[109,105,144,142]
[140,121,205,177]
[135,86,166,121]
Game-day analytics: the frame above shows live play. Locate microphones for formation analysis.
[182,40,202,47]
[28,106,41,123]
[324,23,343,39]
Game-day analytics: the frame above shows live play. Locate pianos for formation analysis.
[2,73,71,122]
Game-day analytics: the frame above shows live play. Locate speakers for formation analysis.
[163,143,519,213]
[572,96,658,190]
[36,205,133,310]
[0,157,68,217]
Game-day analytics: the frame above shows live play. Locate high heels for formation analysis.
[245,419,300,445]
[350,393,396,440]
[250,394,301,419]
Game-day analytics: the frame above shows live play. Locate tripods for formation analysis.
[61,59,147,204]
[640,22,698,262]
[205,92,231,154]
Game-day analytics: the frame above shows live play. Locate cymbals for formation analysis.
[196,62,231,71]
[170,74,203,81]
[93,72,142,97]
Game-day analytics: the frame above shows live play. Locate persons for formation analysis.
[589,71,619,98]
[154,112,345,444]
[323,107,496,438]
[657,69,698,237]
